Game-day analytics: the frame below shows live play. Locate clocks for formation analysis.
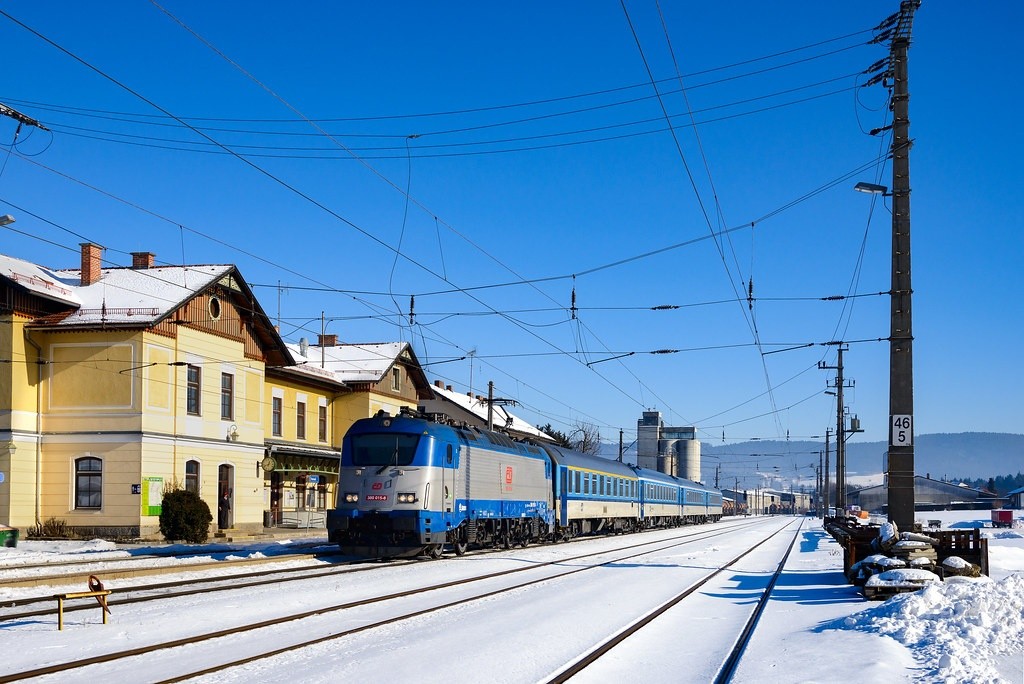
[262,456,277,471]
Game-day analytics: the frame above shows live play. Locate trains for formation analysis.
[323,405,724,562]
